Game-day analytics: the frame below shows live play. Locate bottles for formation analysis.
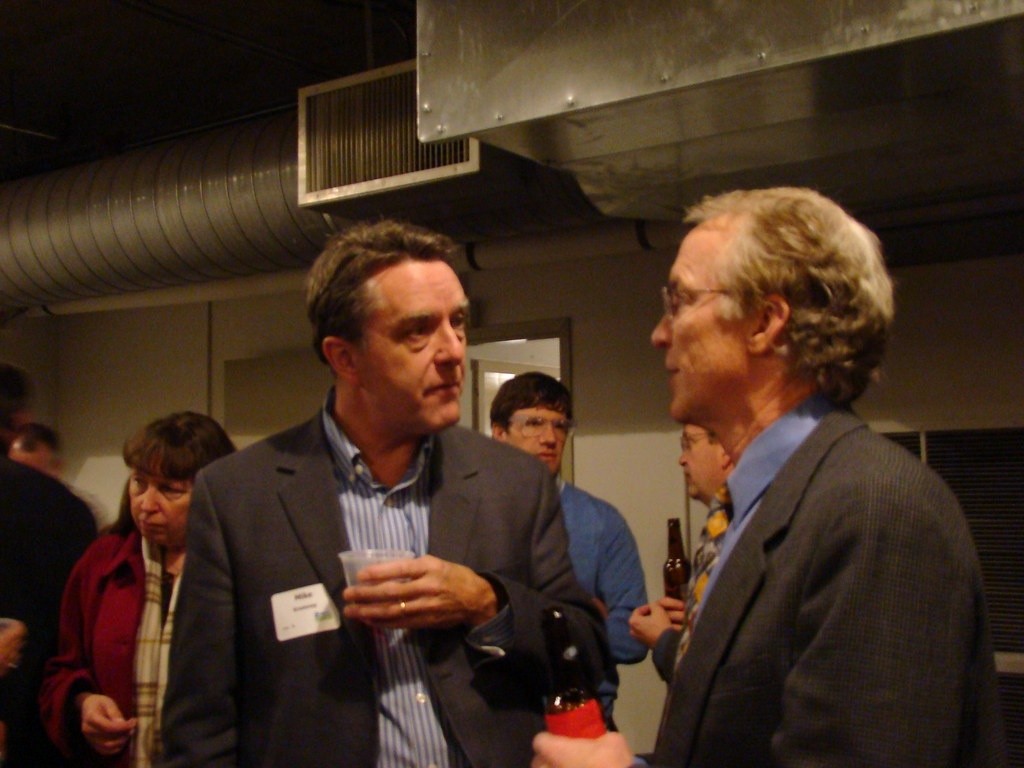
[664,517,691,603]
[536,605,606,739]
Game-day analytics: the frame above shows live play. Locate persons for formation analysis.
[0,362,109,768]
[531,186,1005,768]
[160,221,606,768]
[489,372,650,732]
[629,421,737,686]
[35,411,238,768]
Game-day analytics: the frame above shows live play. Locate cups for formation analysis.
[337,550,416,623]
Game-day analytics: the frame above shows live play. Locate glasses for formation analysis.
[660,285,733,321]
[507,415,574,440]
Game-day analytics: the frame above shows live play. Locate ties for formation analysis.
[674,481,735,669]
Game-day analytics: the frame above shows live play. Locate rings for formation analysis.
[400,601,406,617]
